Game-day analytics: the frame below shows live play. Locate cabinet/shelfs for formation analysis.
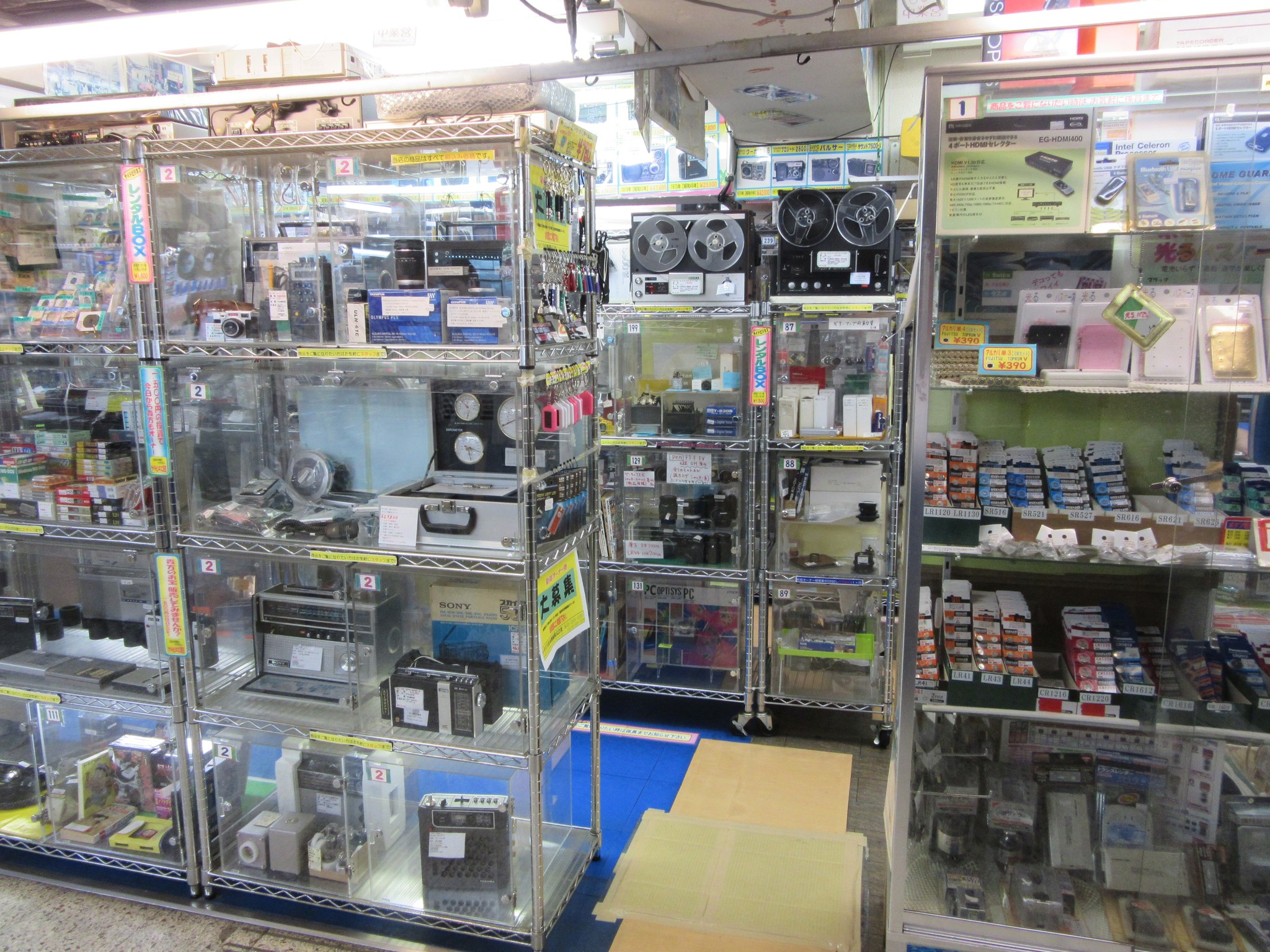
[590,296,758,739]
[867,50,1270,952]
[755,302,912,734]
[0,112,608,952]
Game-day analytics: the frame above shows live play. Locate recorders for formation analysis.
[387,668,488,740]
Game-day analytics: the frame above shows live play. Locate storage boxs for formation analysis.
[447,293,514,343]
[808,458,883,520]
[368,286,448,344]
[916,636,1270,732]
[921,476,1270,550]
[781,378,875,438]
[375,373,577,555]
[977,0,1143,93]
